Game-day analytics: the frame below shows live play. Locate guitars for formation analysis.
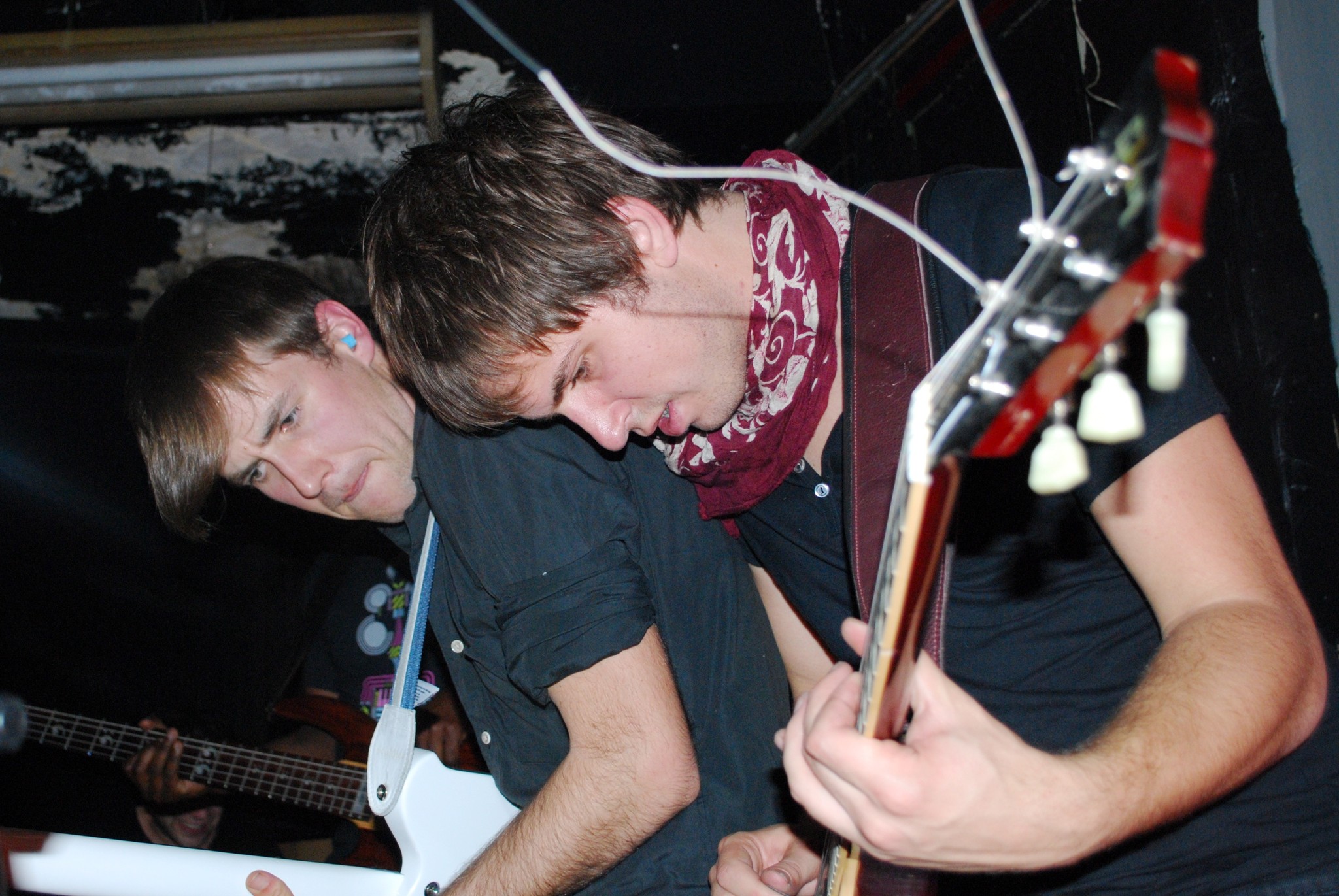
[0,695,493,875]
[812,48,1213,896]
[0,742,524,896]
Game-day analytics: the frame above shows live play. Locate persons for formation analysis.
[125,256,796,896]
[360,90,1339,895]
